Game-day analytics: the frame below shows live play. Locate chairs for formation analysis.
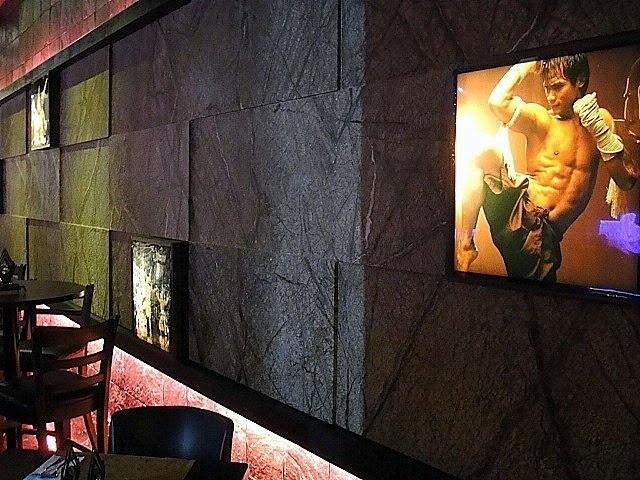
[106,407,235,460]
[1,313,120,455]
[1,248,98,450]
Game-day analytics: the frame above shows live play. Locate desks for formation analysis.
[0,447,249,480]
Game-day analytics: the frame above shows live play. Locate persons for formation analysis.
[455,55,639,284]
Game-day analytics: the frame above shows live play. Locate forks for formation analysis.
[43,459,65,476]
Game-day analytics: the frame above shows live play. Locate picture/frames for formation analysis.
[29,70,59,151]
[130,235,189,359]
